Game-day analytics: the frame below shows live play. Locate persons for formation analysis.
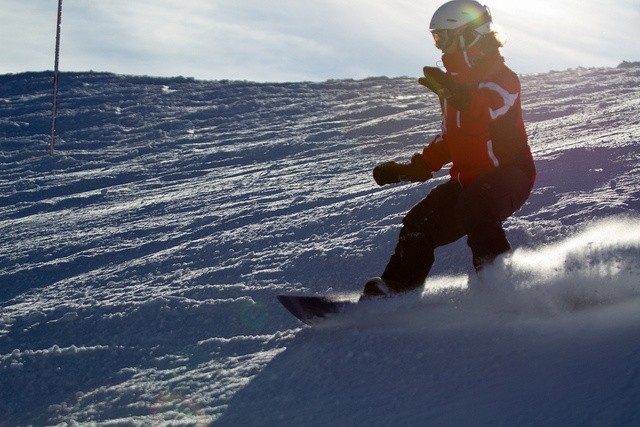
[364,0,536,297]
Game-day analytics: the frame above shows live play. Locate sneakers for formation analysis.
[364,276,396,299]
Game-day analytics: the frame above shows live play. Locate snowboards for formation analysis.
[280,295,360,326]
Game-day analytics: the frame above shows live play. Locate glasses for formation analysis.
[431,12,489,48]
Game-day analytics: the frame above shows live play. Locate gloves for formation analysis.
[373,152,433,185]
[418,66,471,110]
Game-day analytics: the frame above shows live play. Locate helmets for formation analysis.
[430,0,491,48]
